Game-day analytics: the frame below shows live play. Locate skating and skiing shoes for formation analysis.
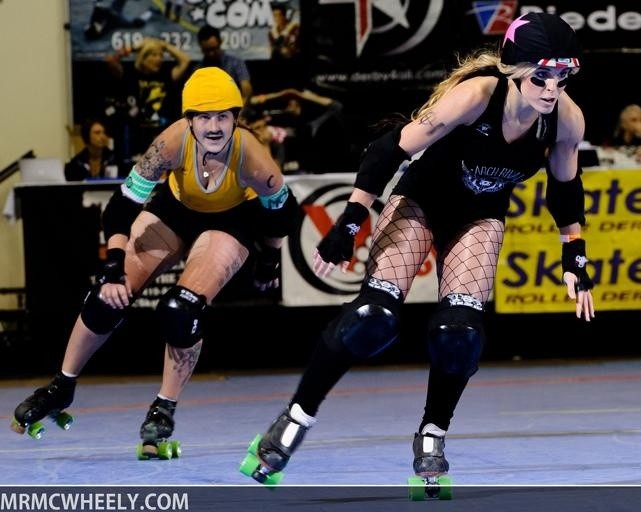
[239,402,317,485]
[10,371,79,441]
[408,422,453,501]
[137,395,182,460]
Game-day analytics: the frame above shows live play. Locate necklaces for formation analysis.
[200,152,224,178]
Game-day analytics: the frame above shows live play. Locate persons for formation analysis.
[193,27,252,105]
[267,0,300,59]
[15,67,304,443]
[106,37,189,159]
[611,103,641,147]
[257,13,595,478]
[249,86,342,173]
[68,118,126,182]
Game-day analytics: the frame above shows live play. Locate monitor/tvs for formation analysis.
[19,158,66,183]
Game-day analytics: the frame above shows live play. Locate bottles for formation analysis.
[105,138,117,181]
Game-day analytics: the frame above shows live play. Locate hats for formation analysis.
[501,10,581,69]
[181,66,243,117]
